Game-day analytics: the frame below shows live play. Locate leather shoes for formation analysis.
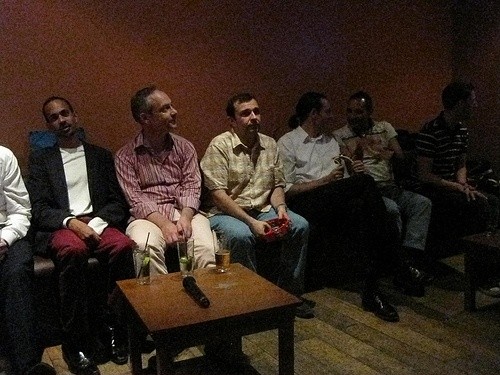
[96,318,128,365]
[400,253,429,285]
[362,293,400,322]
[61,340,101,375]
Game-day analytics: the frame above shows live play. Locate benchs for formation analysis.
[1,130,450,337]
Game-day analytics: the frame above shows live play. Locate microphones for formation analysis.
[183,277,210,308]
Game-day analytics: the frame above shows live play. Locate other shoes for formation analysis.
[472,278,500,297]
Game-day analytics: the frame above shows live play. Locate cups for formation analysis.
[213,229,230,273]
[134,244,150,285]
[176,238,194,276]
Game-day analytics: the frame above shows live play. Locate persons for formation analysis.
[276,93,425,321]
[200,92,313,317]
[415,79,500,296]
[0,144,56,375]
[333,91,432,296]
[114,86,252,368]
[27,96,128,375]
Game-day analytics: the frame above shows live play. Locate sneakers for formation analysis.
[290,301,314,319]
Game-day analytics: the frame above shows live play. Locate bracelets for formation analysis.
[275,203,287,212]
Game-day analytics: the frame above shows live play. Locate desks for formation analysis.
[116,262,305,375]
[460,231,500,312]
